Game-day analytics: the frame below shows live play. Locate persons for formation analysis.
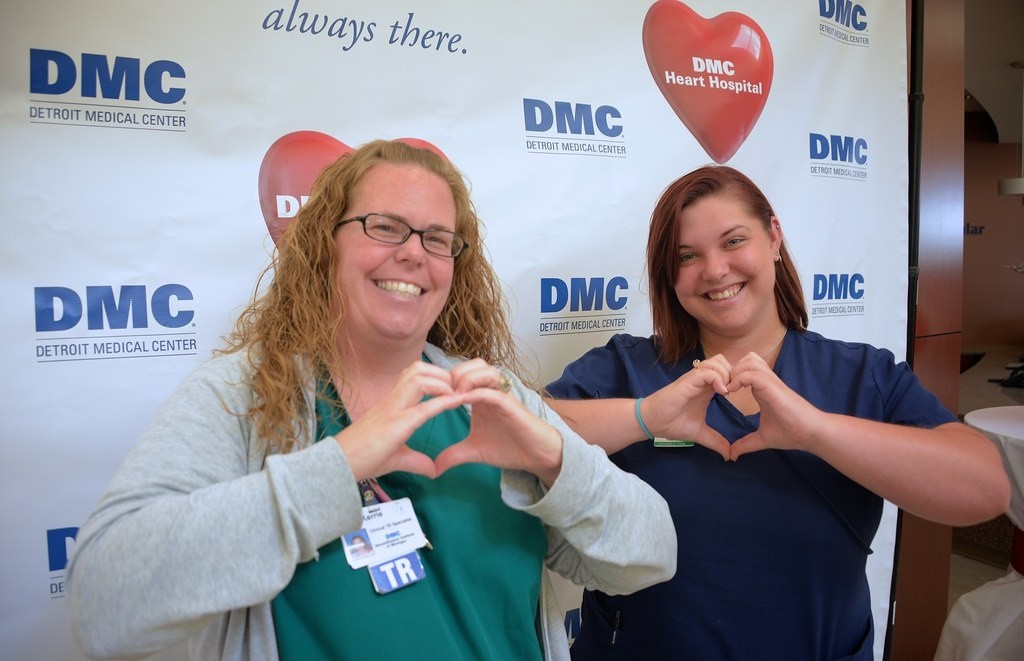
[65,140,678,661]
[537,164,1011,661]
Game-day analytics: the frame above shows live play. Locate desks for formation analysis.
[932,405,1024,661]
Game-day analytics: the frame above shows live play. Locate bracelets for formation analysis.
[635,397,654,440]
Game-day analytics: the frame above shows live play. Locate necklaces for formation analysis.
[700,330,787,368]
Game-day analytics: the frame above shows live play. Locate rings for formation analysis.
[496,373,512,393]
[693,359,700,369]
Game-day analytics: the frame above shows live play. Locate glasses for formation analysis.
[333,213,469,258]
